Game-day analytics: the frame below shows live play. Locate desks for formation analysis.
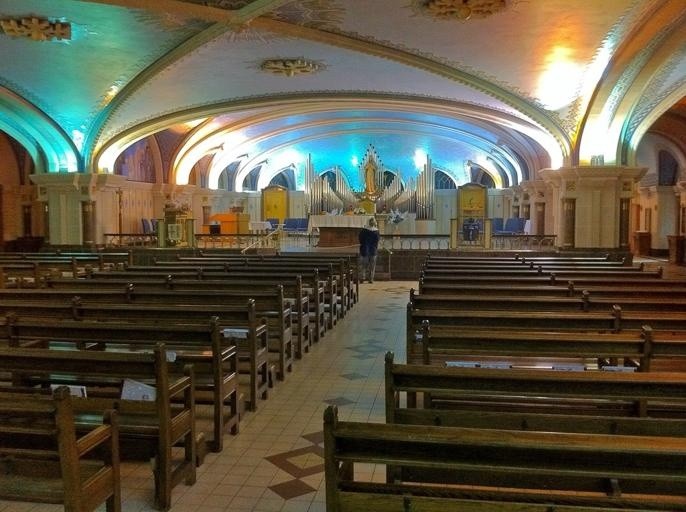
[248,221,272,246]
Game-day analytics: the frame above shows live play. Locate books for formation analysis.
[222,328,248,339]
[120,379,156,402]
[444,361,638,374]
[50,385,87,400]
[47,341,78,382]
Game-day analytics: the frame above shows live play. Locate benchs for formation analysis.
[0,243,685,512]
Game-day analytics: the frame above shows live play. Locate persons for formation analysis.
[359,216,380,284]
[363,155,377,193]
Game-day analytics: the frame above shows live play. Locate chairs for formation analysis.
[461,216,526,250]
[266,218,309,242]
[151,217,160,237]
[142,218,158,246]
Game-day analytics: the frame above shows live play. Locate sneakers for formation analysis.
[368,278,374,283]
[360,278,364,283]
